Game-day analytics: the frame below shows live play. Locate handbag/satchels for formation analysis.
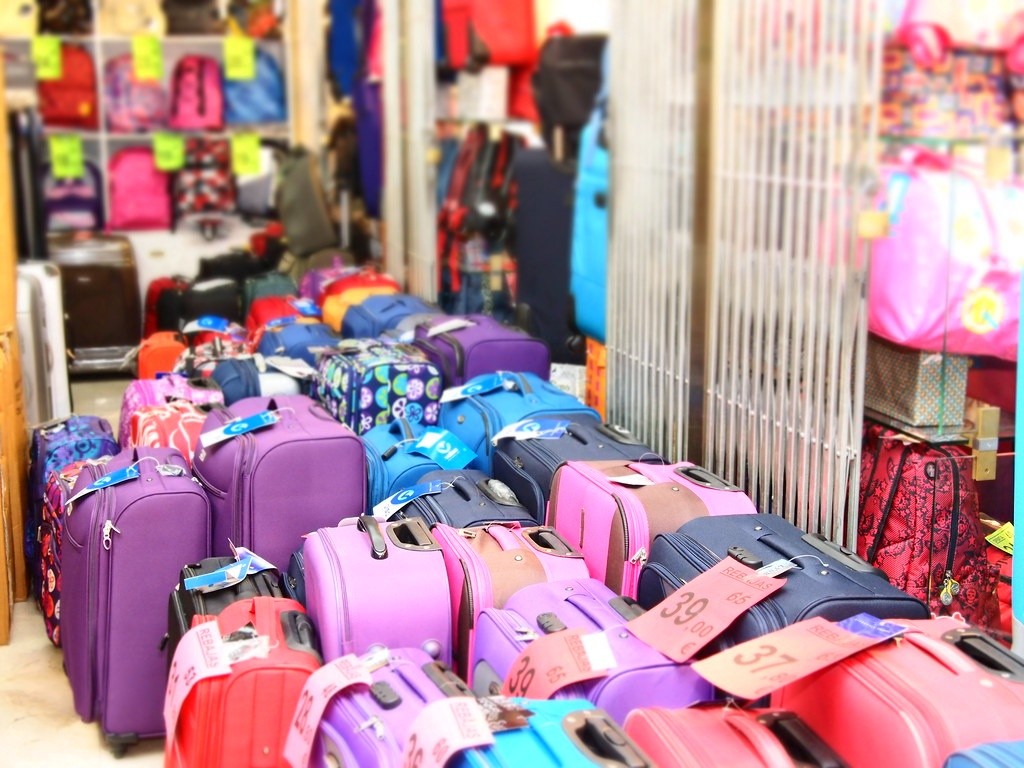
[817,1,1024,424]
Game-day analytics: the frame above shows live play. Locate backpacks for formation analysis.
[847,420,1001,641]
[1,1,287,232]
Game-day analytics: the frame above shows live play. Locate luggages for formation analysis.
[11,216,1022,768]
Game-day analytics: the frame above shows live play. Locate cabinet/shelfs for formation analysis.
[1,0,294,231]
